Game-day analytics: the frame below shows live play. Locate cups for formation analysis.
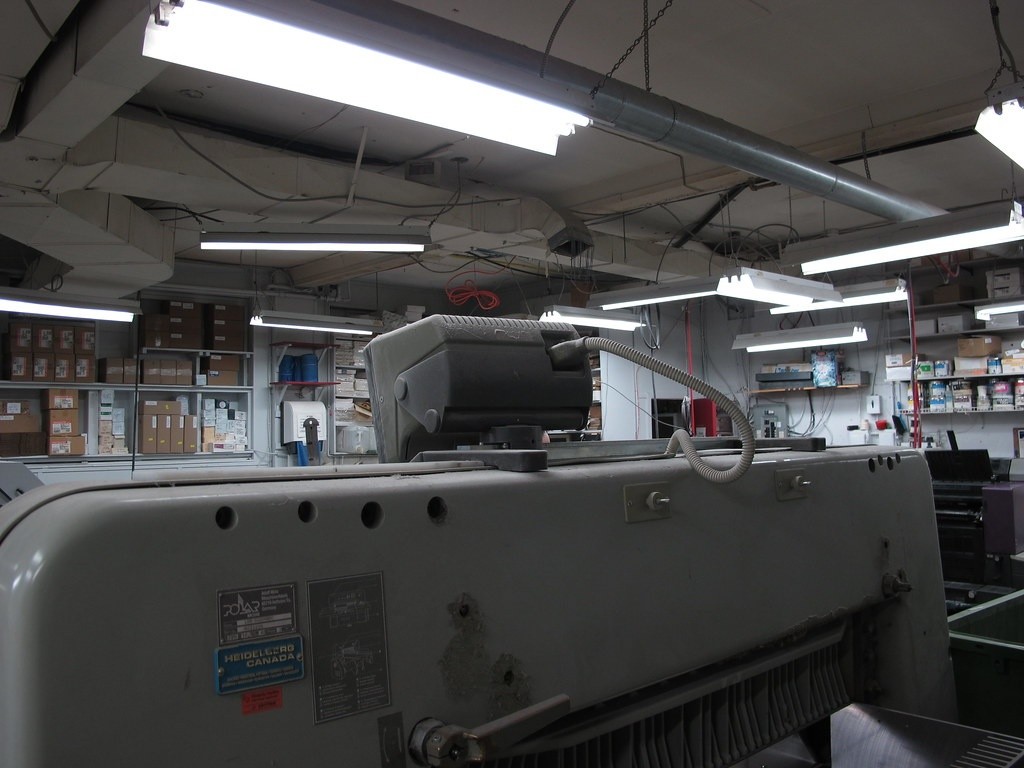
[875,419,887,430]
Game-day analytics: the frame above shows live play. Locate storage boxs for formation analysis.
[335,303,426,454]
[0,300,248,456]
[588,354,601,428]
[755,346,869,393]
[886,249,1024,413]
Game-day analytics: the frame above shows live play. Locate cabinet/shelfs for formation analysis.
[546,326,652,441]
[885,269,1024,459]
[133,288,254,470]
[1,297,139,473]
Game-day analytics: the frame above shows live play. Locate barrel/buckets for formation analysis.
[278,353,318,382]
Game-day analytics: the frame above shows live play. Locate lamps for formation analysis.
[1,270,143,323]
[181,157,487,251]
[251,249,385,336]
[537,0,1024,354]
[141,0,673,157]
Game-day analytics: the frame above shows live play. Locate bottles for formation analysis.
[954,395,969,412]
[907,383,914,410]
[989,378,996,395]
[929,382,945,413]
[945,384,954,412]
